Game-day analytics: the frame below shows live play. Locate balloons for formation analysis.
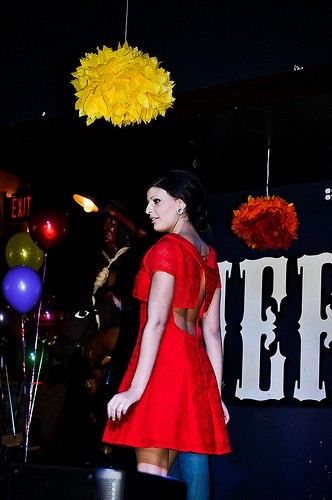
[21,341,49,371]
[3,267,41,316]
[4,232,44,272]
[36,310,60,326]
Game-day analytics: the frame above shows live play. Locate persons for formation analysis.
[87,210,141,456]
[102,180,230,500]
